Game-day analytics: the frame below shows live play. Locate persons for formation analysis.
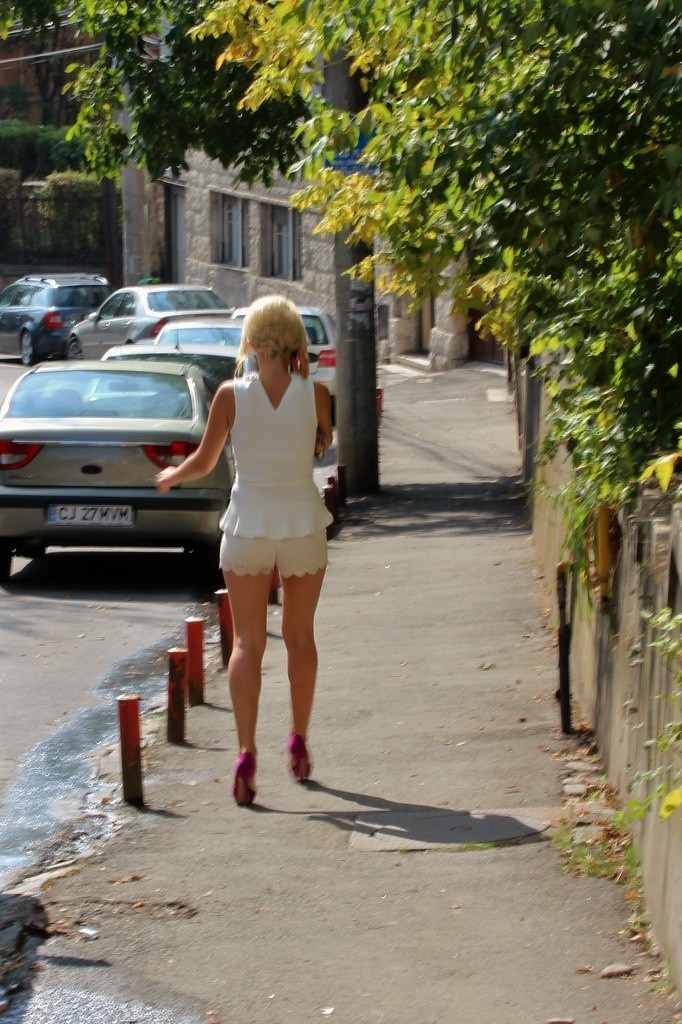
[155,295,333,803]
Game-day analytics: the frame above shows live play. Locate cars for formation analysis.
[65,284,338,402]
[0,360,234,595]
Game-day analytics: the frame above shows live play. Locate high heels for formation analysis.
[288,735,310,784]
[232,752,257,806]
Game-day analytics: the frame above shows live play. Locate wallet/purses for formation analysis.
[314,424,326,461]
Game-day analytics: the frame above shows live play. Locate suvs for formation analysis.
[0,274,112,366]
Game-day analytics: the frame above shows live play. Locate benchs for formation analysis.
[45,388,188,416]
